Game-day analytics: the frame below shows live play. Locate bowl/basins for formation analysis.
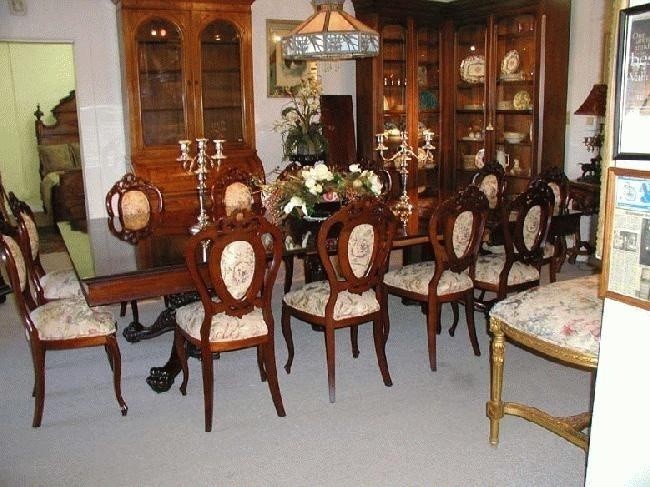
[503,131,525,144]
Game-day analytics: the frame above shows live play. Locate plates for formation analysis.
[419,91,438,109]
[393,104,406,111]
[475,148,484,169]
[501,49,520,73]
[459,54,486,84]
[513,90,531,110]
[464,101,511,110]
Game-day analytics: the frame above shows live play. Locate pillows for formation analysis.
[37,142,74,176]
[70,142,81,167]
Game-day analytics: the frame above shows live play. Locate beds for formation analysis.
[33,89,88,233]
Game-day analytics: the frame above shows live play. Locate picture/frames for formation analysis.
[265,17,321,98]
[613,2,650,163]
[598,166,650,312]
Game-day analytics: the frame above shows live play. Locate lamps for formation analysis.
[574,84,607,183]
[276,1,382,61]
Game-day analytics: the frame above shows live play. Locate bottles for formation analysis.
[383,72,407,86]
[428,64,439,90]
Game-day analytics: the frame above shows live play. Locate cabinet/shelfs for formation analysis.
[357,0,572,198]
